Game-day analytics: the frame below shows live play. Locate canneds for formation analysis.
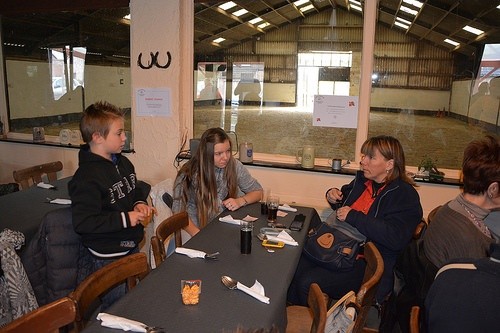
[239,142,253,162]
[33,126,45,141]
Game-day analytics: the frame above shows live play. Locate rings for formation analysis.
[328,197,331,200]
[231,205,234,208]
[337,216,339,219]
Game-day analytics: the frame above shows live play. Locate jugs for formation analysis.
[296,146,316,169]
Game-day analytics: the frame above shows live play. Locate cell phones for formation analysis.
[289,214,306,231]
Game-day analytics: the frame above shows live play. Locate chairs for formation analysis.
[0,160,426,333]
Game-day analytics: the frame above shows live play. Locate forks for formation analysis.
[223,277,237,288]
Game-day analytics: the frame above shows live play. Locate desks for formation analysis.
[83,202,322,333]
[0,175,74,236]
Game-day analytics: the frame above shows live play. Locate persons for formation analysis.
[68,102,157,312]
[167,127,264,256]
[424,136,499,268]
[287,135,423,309]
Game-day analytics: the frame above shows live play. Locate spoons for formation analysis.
[222,276,237,289]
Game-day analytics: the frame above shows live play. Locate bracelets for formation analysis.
[241,196,248,204]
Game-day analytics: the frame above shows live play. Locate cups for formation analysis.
[240,220,253,255]
[33,127,44,142]
[239,142,253,162]
[122,131,130,150]
[332,159,341,170]
[266,196,279,223]
[260,187,271,214]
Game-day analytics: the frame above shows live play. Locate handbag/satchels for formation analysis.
[302,208,368,272]
[323,290,362,333]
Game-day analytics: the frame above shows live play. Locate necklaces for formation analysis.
[372,185,376,197]
[458,201,490,235]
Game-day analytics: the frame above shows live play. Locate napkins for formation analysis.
[219,215,249,226]
[278,203,297,212]
[175,247,206,259]
[96,313,149,333]
[265,230,299,246]
[236,280,271,304]
